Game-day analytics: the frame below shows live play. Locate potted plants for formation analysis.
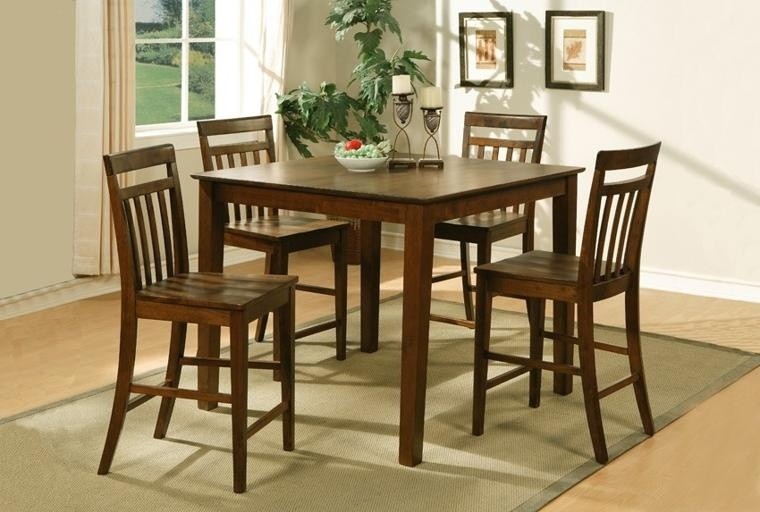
[275,2,436,266]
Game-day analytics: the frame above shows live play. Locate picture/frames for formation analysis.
[458,12,514,88]
[546,11,604,92]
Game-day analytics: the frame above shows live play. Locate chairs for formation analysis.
[98,289,100,293]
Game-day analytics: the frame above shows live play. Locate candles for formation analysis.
[385,75,445,172]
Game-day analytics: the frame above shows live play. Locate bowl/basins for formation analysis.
[334,154,388,173]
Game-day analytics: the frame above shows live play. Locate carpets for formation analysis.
[3,292,759,512]
